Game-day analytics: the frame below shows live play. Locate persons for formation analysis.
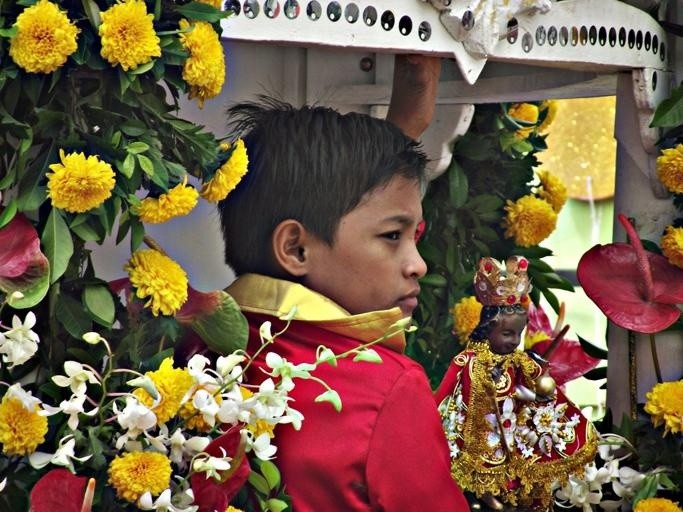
[433,258,598,511]
[173,93,471,512]
